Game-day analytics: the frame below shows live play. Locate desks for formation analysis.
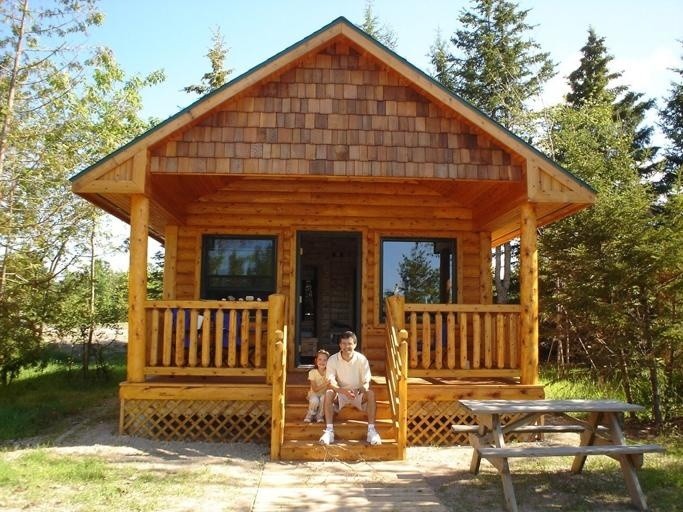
[458,394,649,511]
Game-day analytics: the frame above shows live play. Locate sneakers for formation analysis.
[304,415,313,422]
[367,431,381,444]
[319,427,335,444]
[316,415,324,423]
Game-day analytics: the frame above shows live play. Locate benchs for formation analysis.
[447,424,613,434]
[476,443,669,459]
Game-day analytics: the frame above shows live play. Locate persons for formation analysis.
[302,347,338,423]
[319,330,384,446]
